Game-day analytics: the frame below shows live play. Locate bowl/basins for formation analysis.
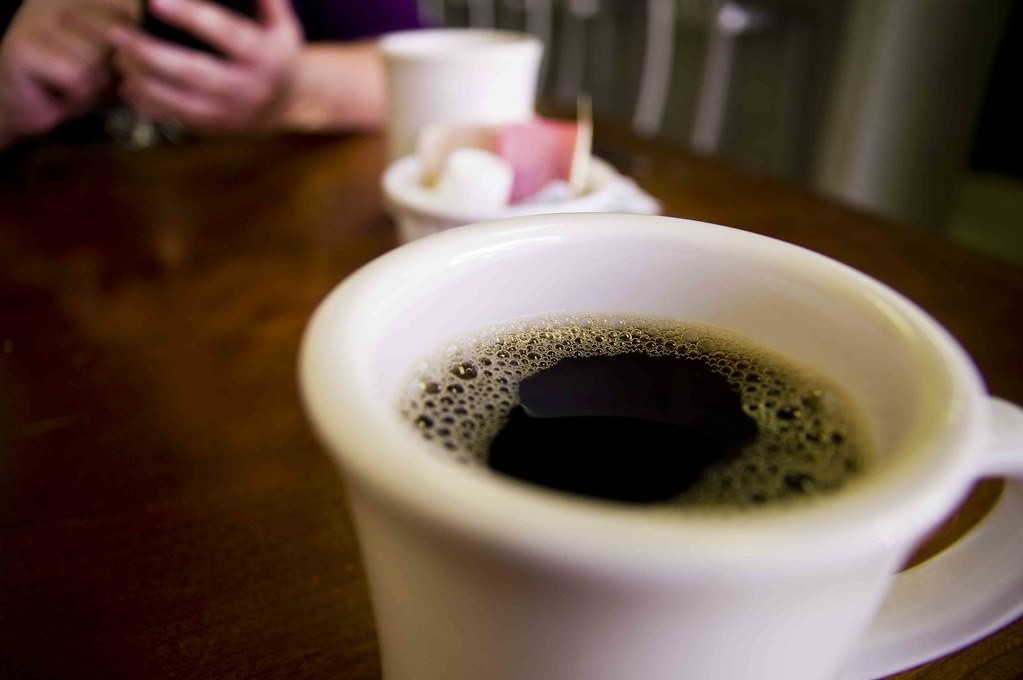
[383,154,618,250]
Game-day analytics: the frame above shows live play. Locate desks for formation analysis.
[3,103,1022,680]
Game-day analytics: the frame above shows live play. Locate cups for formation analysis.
[296,212,1023,680]
[381,27,544,217]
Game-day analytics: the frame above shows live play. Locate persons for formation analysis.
[0,0,418,137]
